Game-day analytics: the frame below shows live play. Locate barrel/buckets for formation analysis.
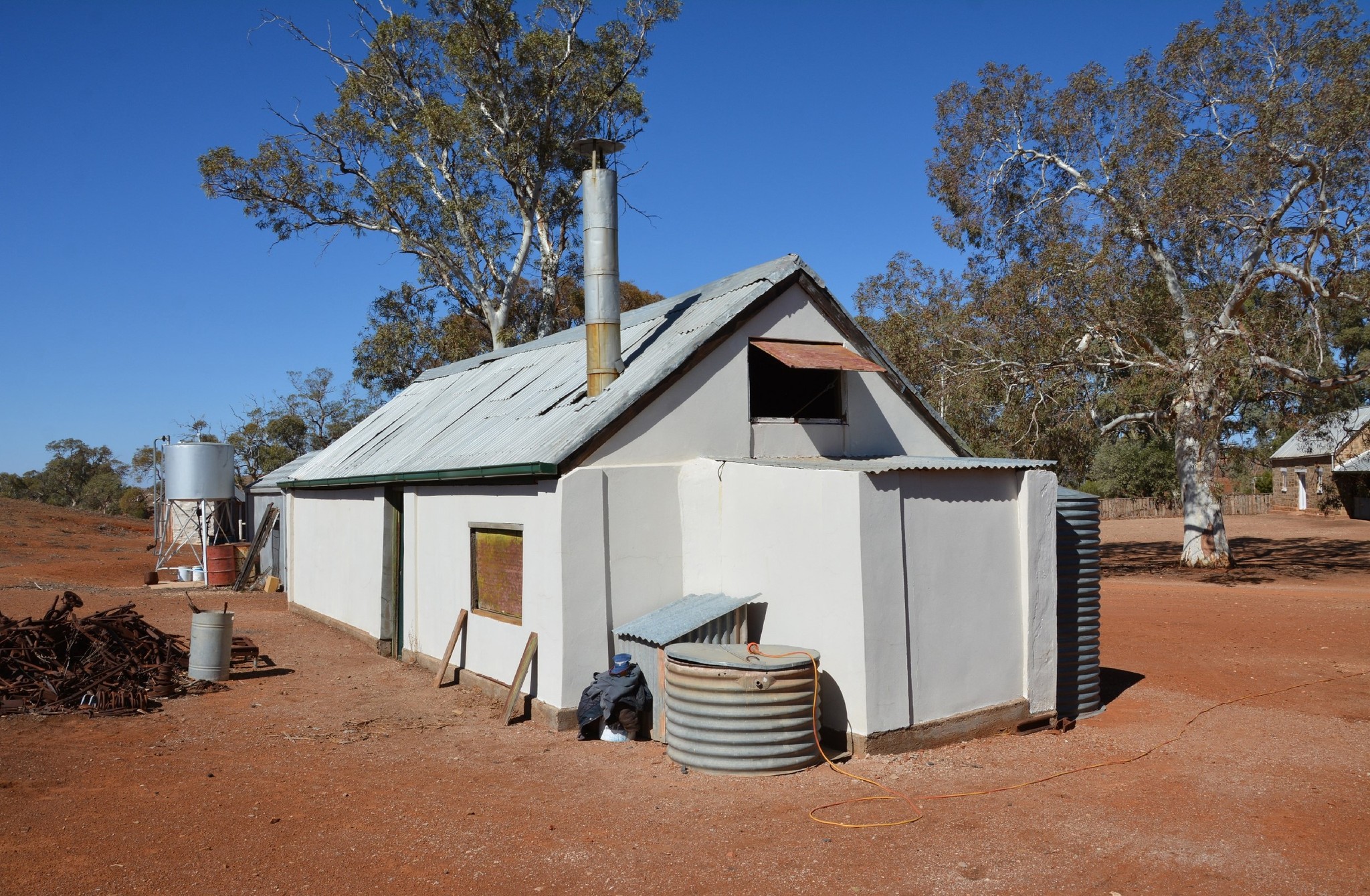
[144,572,159,585]
[191,565,204,581]
[188,609,235,682]
[226,542,256,583]
[598,715,629,742]
[178,566,191,582]
[206,544,236,586]
